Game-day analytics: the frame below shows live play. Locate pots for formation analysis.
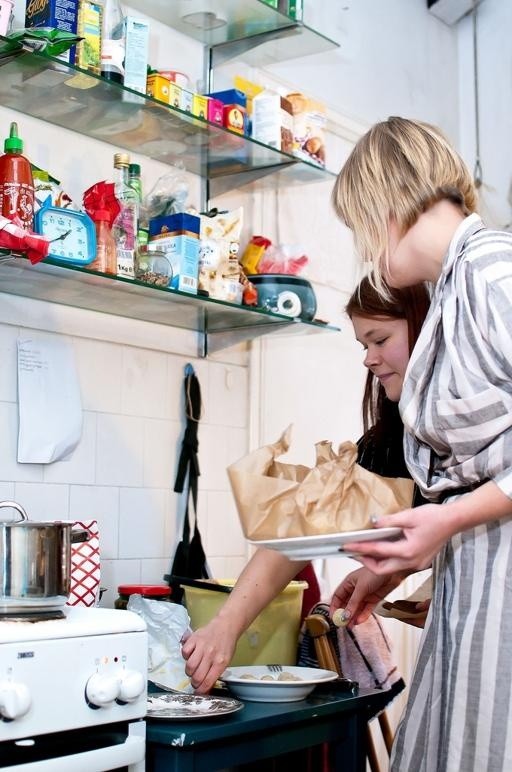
[0,498,75,619]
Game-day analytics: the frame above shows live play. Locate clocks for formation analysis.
[34,207,97,267]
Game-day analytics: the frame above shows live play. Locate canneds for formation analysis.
[115,585,172,610]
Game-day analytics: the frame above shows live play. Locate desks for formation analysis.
[146,686,393,772]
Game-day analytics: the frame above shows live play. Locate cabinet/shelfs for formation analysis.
[0,1,340,356]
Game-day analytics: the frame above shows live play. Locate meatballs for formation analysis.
[238,671,304,682]
[331,608,350,628]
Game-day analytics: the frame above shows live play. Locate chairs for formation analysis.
[305,614,393,771]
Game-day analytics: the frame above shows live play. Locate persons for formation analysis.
[181,270,432,697]
[328,116,510,771]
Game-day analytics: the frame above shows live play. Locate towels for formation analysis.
[297,602,406,721]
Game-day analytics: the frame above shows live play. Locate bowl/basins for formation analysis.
[219,664,339,704]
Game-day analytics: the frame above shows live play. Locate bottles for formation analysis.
[0,118,35,250]
[101,0,124,86]
[110,150,150,283]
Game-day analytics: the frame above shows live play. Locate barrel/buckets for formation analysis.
[162,572,310,666]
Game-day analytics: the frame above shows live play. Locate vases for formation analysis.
[250,273,317,321]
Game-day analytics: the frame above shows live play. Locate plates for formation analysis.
[247,524,401,564]
[146,690,246,721]
